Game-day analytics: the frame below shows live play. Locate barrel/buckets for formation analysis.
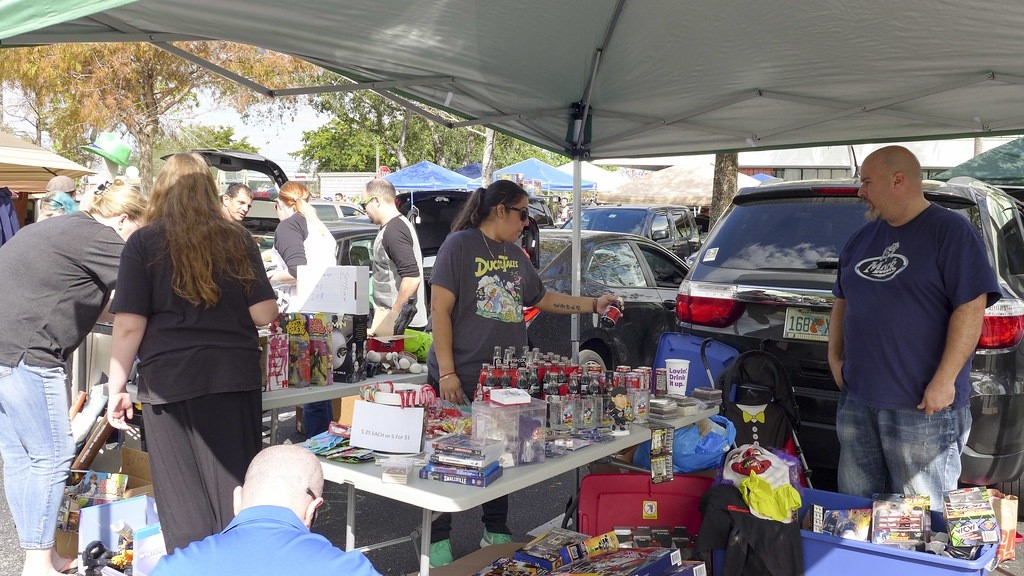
[360,381,432,457]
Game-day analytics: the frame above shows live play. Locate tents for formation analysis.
[0,130,99,192]
[0,0,1024,532]
[557,134,1024,206]
[380,158,631,228]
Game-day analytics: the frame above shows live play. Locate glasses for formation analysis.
[508,207,530,222]
[360,195,378,209]
[307,486,320,527]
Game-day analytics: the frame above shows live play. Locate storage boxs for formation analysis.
[420,390,1001,576]
[56,446,165,576]
[295,264,370,315]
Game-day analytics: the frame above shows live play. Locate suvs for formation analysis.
[675,176,1024,528]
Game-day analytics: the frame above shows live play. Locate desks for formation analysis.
[260,370,428,447]
[55,406,721,576]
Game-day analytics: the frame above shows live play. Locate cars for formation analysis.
[60,148,704,396]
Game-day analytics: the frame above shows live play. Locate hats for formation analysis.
[46,176,77,193]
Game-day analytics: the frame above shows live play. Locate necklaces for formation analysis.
[481,225,506,271]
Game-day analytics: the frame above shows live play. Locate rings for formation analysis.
[451,395,456,399]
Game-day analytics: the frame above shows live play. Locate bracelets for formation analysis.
[593,299,598,314]
[439,370,456,381]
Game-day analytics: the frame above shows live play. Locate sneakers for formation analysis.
[430,539,454,568]
[480,528,513,549]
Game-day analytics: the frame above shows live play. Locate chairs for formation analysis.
[348,245,372,272]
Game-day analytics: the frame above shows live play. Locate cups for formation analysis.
[666,360,691,396]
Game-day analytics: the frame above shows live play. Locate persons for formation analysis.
[393,296,418,335]
[427,180,624,569]
[360,179,428,337]
[36,176,80,222]
[324,193,345,202]
[219,182,271,438]
[81,474,98,508]
[828,145,1002,513]
[147,445,384,576]
[267,180,338,439]
[0,178,149,576]
[107,151,279,556]
[555,197,570,222]
[608,386,627,431]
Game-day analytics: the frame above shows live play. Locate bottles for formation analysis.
[598,298,623,329]
[479,344,615,435]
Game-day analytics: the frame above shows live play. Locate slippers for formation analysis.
[58,556,77,574]
